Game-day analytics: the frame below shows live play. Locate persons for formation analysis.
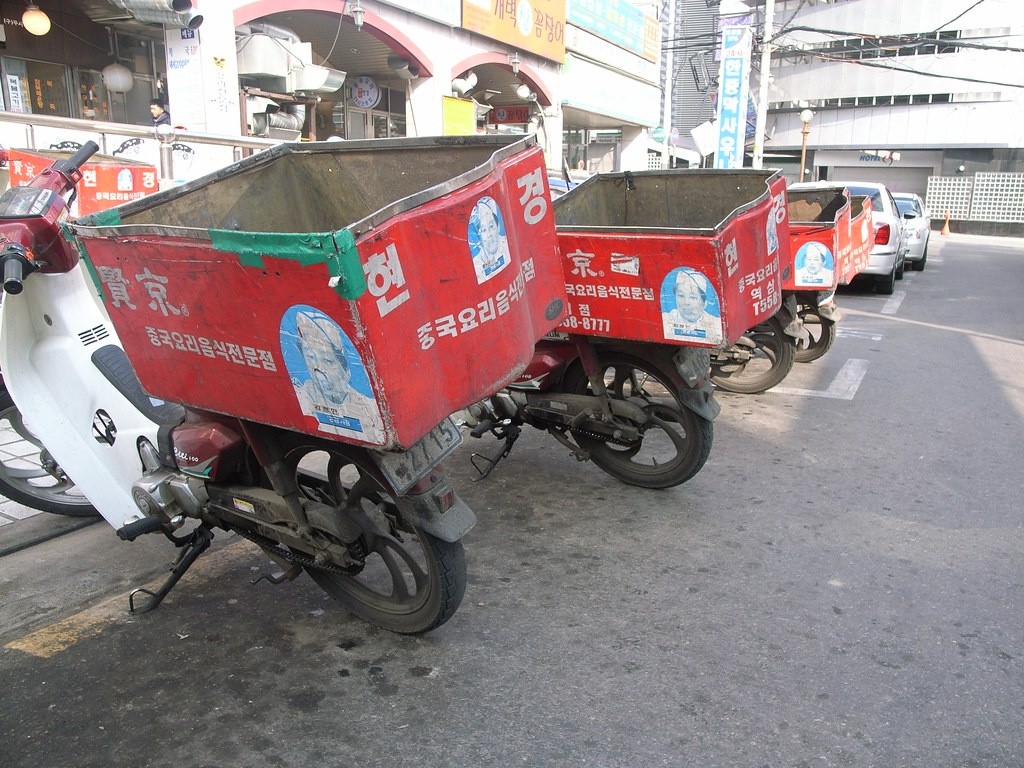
[795,244,834,287]
[662,270,723,345]
[294,312,383,443]
[149,99,171,127]
[472,198,512,285]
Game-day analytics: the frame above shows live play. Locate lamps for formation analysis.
[506,51,521,77]
[352,0,365,32]
[22,0,51,35]
[101,26,134,92]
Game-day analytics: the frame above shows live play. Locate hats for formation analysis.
[808,242,827,262]
[296,312,342,350]
[677,269,706,292]
[478,199,497,216]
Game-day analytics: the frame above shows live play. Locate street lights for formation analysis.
[798,108,813,183]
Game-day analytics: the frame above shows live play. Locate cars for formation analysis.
[787,180,916,296]
[877,192,933,271]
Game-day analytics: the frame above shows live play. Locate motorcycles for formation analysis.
[706,183,876,396]
[0,136,573,635]
[324,133,782,489]
